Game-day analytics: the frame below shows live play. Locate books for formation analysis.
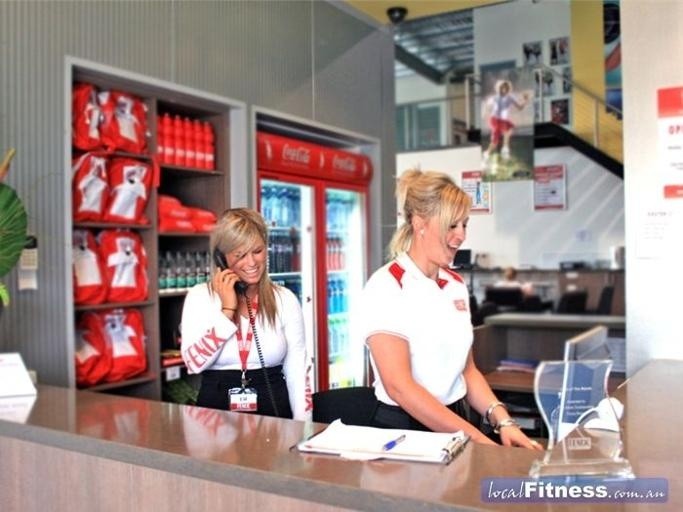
[497,366,534,373]
[499,356,540,369]
[297,417,466,464]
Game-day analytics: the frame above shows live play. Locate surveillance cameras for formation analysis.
[386,7,408,24]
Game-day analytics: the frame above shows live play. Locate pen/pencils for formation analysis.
[384,435,406,450]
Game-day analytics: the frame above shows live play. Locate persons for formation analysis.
[180,207,310,422]
[358,441,474,503]
[493,266,535,296]
[482,80,530,162]
[360,163,542,451]
[181,404,304,473]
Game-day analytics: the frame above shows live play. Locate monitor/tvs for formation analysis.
[555,323,609,445]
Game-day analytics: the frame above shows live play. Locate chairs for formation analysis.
[311,386,378,426]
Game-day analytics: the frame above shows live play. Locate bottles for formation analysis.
[157,250,211,289]
[324,190,359,390]
[260,184,302,274]
[155,113,216,171]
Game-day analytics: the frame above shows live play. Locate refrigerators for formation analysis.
[255,130,376,393]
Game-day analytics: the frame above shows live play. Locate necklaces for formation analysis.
[236,292,253,304]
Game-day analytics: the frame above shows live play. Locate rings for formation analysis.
[530,438,537,445]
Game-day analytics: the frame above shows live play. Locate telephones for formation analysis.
[208,245,248,294]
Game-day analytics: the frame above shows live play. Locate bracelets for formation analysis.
[485,401,508,427]
[221,306,237,312]
[493,418,521,433]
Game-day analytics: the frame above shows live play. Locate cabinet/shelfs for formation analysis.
[64,54,248,403]
